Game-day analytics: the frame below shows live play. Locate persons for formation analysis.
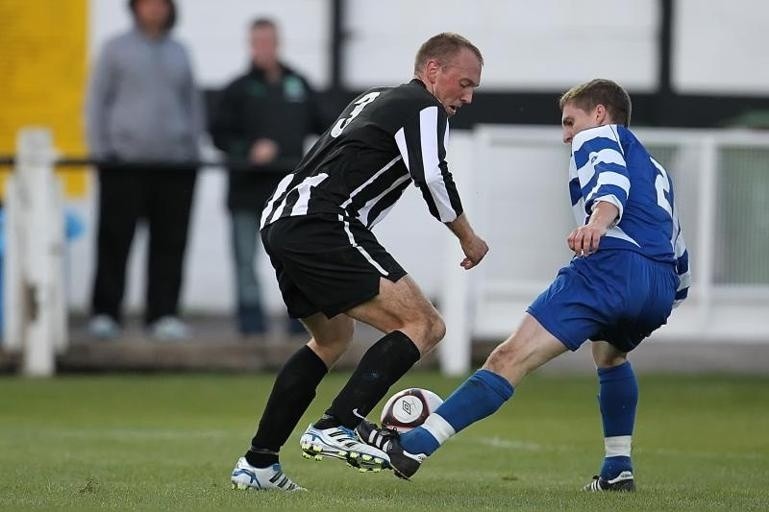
[212,20,337,334]
[231,32,489,493]
[87,0,199,341]
[356,78,691,493]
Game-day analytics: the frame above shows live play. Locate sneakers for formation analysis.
[580,470,634,491]
[353,419,420,481]
[231,457,306,492]
[300,419,391,480]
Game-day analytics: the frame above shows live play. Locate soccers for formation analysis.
[380,388,444,434]
